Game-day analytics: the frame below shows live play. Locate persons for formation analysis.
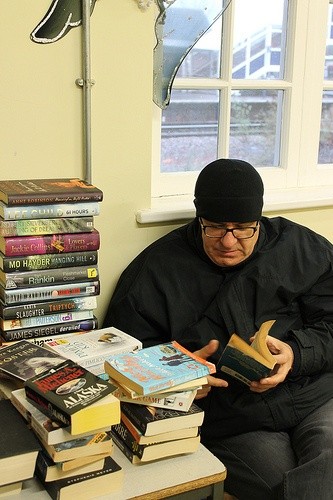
[159,353,185,362]
[102,159,333,500]
[162,357,194,367]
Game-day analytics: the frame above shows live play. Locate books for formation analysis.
[0,178,216,500]
[216,319,276,388]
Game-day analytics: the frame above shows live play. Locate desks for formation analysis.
[0,439,227,500]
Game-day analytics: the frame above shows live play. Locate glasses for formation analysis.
[197,218,260,239]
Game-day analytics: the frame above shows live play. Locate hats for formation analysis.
[193,158,264,222]
